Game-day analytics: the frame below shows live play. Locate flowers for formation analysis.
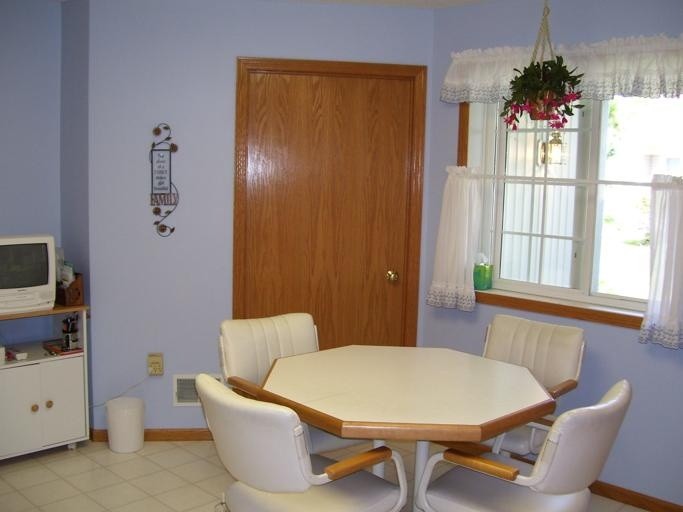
[499,55,585,164]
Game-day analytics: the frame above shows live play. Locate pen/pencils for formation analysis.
[63,314,79,333]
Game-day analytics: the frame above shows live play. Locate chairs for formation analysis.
[415,375,632,512]
[218,313,386,479]
[195,374,408,512]
[478,313,586,458]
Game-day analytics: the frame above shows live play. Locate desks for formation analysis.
[260,344,557,512]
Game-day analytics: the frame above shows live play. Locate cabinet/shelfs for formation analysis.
[0,304,91,461]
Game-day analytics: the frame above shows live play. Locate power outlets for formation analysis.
[146,353,163,376]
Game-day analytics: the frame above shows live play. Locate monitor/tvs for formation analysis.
[0,235,56,316]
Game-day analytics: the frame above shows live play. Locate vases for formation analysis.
[530,100,551,120]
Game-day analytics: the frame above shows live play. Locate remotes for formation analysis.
[5,347,28,361]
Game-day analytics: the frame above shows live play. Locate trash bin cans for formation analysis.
[106,397,145,454]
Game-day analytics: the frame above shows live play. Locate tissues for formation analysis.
[474,253,493,291]
